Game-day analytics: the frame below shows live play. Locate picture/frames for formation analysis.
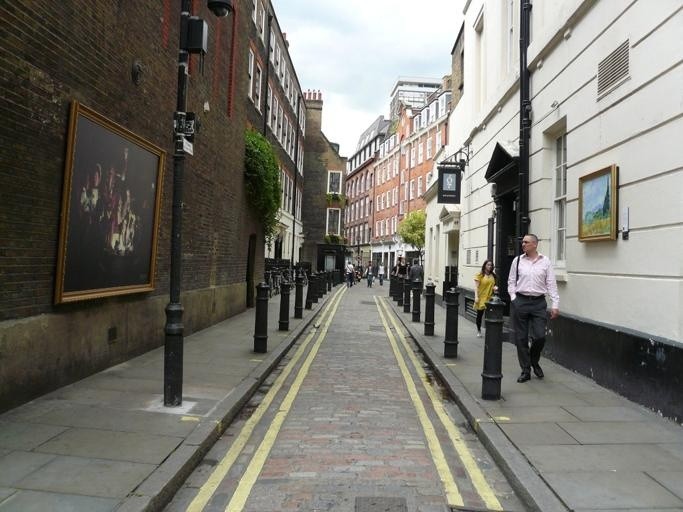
[54,97,167,306]
[577,163,619,242]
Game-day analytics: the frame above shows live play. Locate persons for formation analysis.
[392,256,423,294]
[473,260,498,337]
[364,261,375,288]
[507,234,559,382]
[345,261,354,287]
[377,263,385,285]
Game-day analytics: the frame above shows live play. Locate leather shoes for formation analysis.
[517,372,530,382]
[531,361,543,377]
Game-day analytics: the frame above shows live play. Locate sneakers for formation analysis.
[477,330,482,338]
